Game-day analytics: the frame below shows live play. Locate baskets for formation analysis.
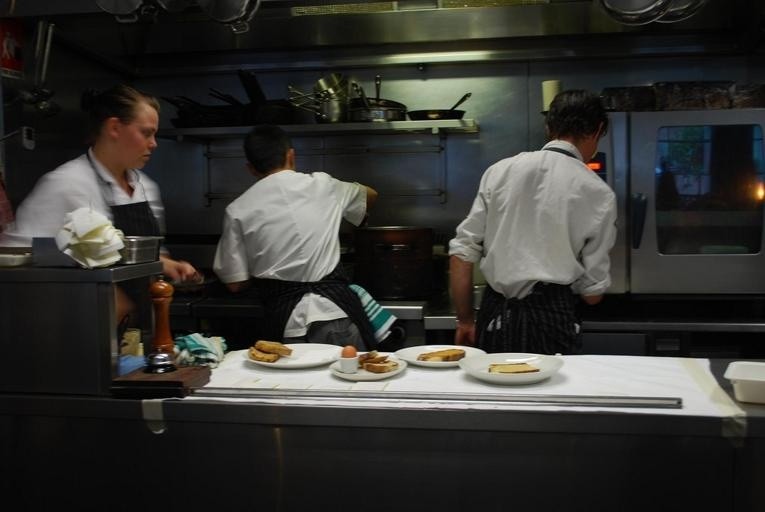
[653,81,762,110]
[601,86,654,111]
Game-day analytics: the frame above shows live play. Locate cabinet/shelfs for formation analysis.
[159,119,481,213]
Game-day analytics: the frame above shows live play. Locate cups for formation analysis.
[335,355,360,375]
[119,327,141,357]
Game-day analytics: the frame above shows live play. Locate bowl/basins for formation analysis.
[720,360,765,405]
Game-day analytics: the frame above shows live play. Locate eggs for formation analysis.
[342,345,357,358]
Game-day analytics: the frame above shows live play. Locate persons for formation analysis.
[213,126,378,350]
[448,89,617,354]
[0,86,203,283]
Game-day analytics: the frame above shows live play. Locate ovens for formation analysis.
[536,78,764,302]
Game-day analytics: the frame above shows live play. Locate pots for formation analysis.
[151,67,474,123]
[147,0,265,35]
[349,222,441,302]
[653,1,710,24]
[601,0,674,27]
[93,0,147,23]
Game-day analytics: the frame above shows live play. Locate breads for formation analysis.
[417,349,465,361]
[358,350,399,373]
[248,340,292,362]
[489,363,540,373]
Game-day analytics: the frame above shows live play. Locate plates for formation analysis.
[455,351,566,386]
[241,342,349,371]
[395,344,484,370]
[327,351,408,381]
